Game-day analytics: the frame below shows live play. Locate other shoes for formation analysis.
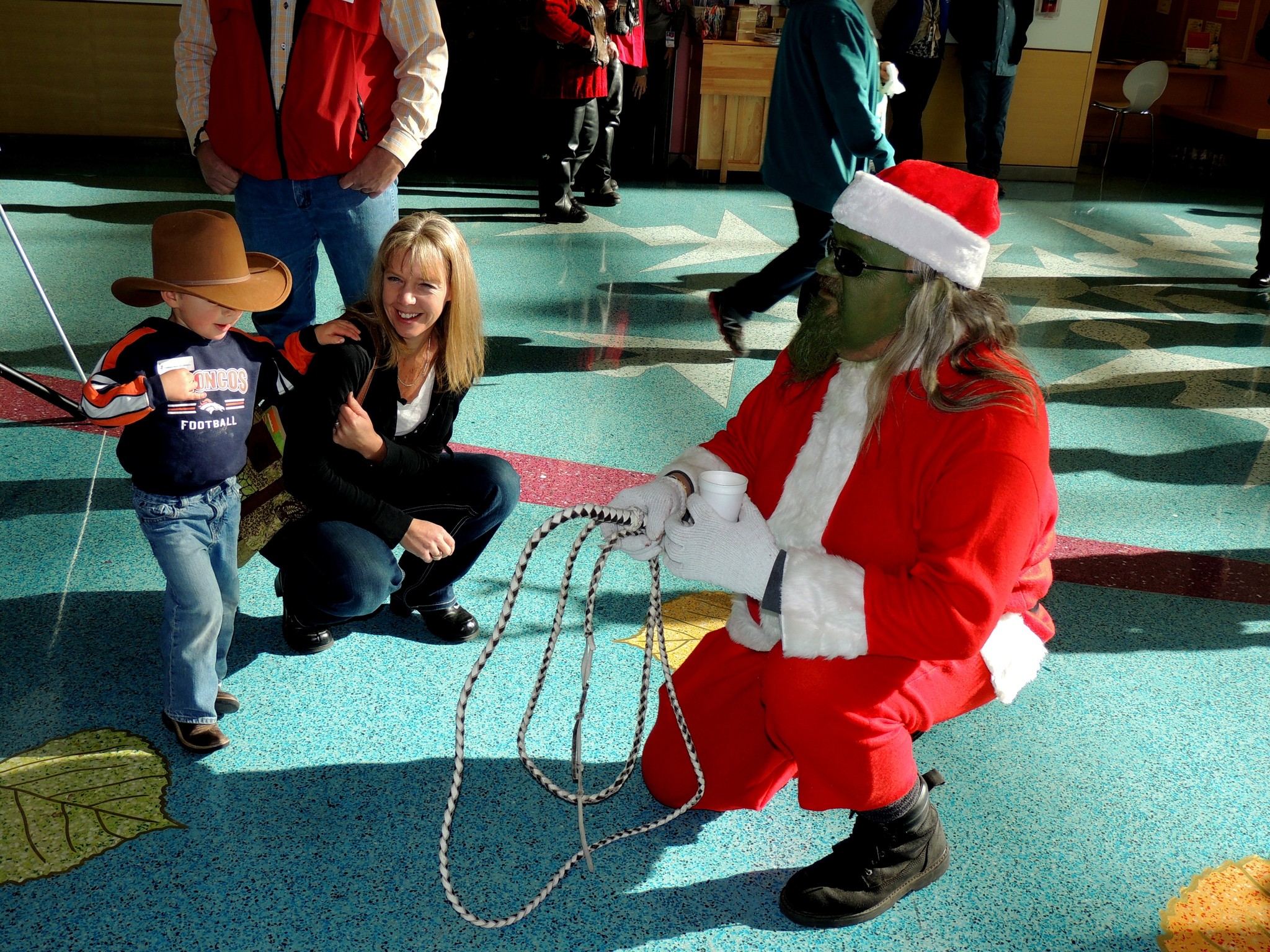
[162,707,229,753]
[571,197,586,210]
[591,192,619,207]
[586,177,618,189]
[214,687,239,714]
[566,208,589,223]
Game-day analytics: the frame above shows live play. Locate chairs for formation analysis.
[1089,60,1170,186]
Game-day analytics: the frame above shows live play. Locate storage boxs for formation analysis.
[723,4,786,41]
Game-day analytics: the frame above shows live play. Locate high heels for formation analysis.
[274,569,334,654]
[388,590,480,642]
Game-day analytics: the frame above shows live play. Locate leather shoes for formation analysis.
[1250,269,1270,287]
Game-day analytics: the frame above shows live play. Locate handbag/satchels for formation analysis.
[605,5,630,37]
[548,6,597,66]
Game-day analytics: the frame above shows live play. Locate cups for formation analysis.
[698,470,749,523]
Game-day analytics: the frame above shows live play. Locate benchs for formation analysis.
[1160,103,1270,196]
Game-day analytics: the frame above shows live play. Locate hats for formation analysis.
[110,205,292,313]
[830,159,1000,291]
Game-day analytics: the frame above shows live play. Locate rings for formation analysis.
[334,420,340,428]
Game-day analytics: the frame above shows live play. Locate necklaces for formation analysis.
[398,333,432,386]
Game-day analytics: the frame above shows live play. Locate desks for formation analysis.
[682,34,779,184]
[1085,62,1228,146]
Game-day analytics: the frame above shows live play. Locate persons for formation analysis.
[171,0,451,333]
[275,211,523,653]
[710,1,1034,360]
[77,206,364,750]
[540,0,650,223]
[602,162,1060,931]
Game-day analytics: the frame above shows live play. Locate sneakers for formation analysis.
[780,769,949,925]
[709,291,746,356]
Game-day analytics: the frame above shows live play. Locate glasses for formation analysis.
[823,229,917,278]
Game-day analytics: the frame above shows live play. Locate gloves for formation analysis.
[661,493,782,604]
[600,474,688,563]
